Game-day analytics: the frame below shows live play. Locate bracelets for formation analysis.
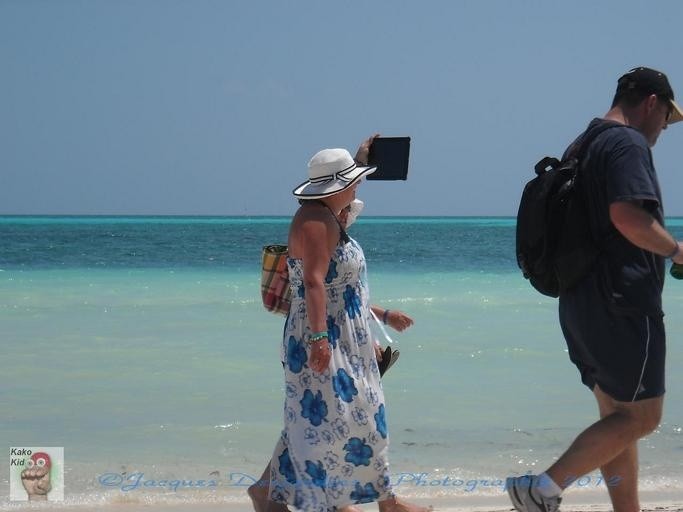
[351,155,367,166]
[663,238,680,259]
[308,331,329,344]
[381,308,391,327]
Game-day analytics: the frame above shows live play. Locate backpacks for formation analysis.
[515,120,631,298]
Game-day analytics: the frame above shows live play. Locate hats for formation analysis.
[293,148,376,201]
[346,198,364,229]
[617,68,683,124]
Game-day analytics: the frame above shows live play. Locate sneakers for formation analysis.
[506,474,564,512]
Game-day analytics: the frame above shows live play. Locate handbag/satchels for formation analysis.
[261,244,292,316]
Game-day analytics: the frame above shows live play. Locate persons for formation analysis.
[503,65,681,511]
[265,130,416,512]
[245,149,442,512]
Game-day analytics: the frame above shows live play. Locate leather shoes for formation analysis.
[375,346,399,378]
